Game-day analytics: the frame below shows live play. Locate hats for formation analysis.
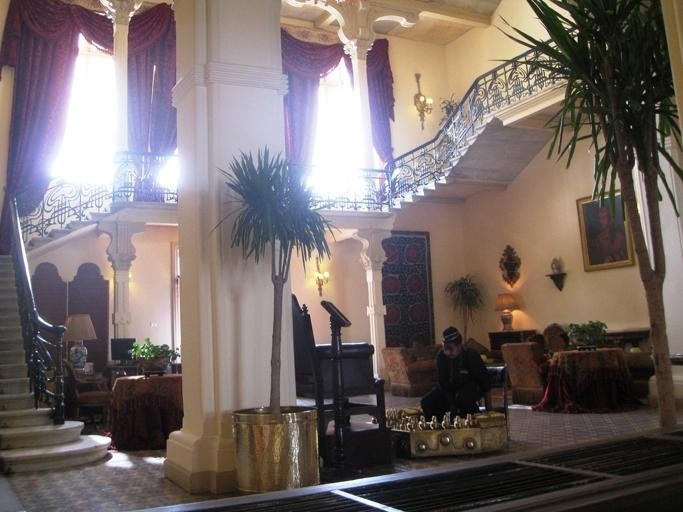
[442,326,460,343]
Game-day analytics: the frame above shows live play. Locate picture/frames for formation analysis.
[577,190,635,272]
[499,245,520,289]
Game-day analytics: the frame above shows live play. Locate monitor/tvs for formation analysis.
[110,337,135,364]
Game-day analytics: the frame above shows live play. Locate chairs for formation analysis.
[62,359,114,432]
[476,365,512,442]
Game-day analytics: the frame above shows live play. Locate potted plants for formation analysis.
[569,321,607,345]
[209,146,340,493]
[128,338,179,373]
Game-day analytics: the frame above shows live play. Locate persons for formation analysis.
[419,326,490,425]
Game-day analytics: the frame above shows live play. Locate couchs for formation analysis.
[291,293,394,479]
[380,331,654,405]
[107,374,184,450]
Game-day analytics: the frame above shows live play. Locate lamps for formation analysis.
[63,314,97,370]
[495,293,519,331]
[315,257,329,296]
[414,74,433,131]
